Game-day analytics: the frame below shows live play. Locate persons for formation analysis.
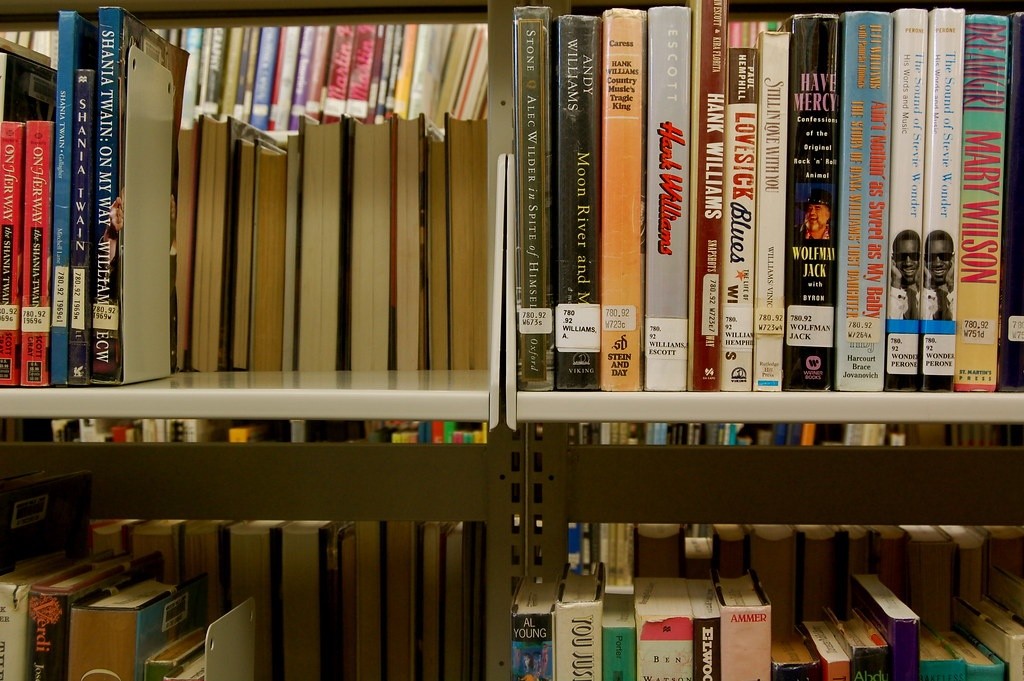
[794,188,831,240]
[923,230,955,320]
[889,230,922,320]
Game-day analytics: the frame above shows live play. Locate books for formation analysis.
[514,4,1024,392]
[0,417,487,681]
[510,423,1024,679]
[1,0,490,387]
